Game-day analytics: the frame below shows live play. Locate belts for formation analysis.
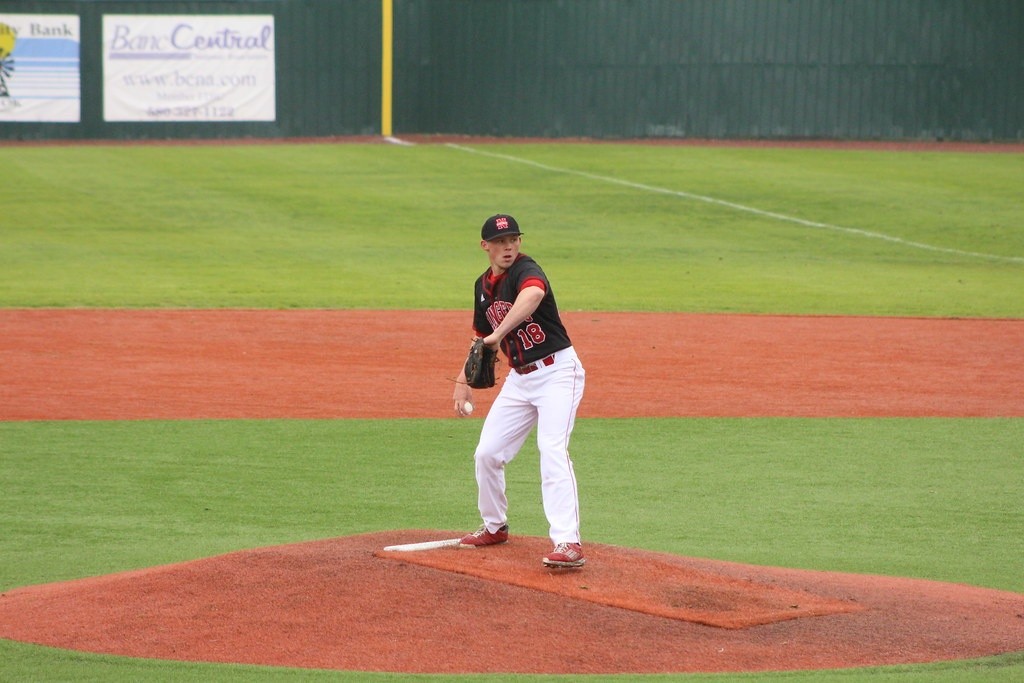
[515,355,555,375]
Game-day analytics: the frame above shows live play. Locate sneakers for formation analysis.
[459,523,508,548]
[542,543,586,567]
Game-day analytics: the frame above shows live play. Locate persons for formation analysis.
[453,215,586,566]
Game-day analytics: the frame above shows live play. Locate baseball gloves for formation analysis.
[444,337,501,390]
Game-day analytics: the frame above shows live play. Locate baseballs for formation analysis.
[459,400,476,417]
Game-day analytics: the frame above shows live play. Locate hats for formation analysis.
[481,214,524,241]
